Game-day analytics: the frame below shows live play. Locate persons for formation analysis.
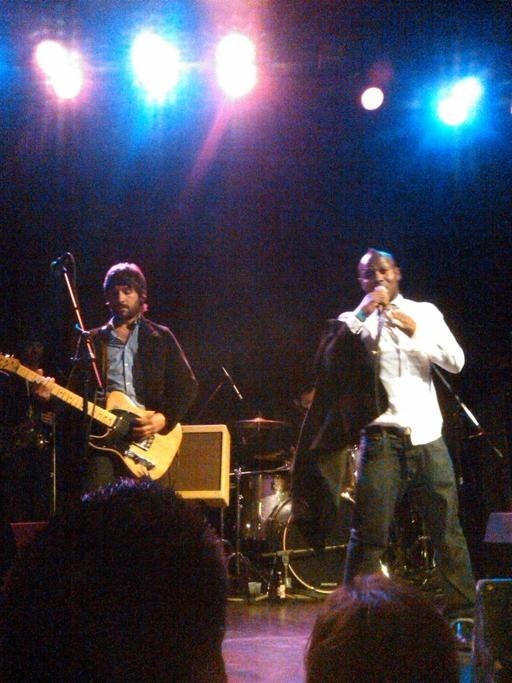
[0,467,230,681]
[244,381,317,462]
[305,573,461,682]
[0,331,69,522]
[333,249,477,649]
[29,261,200,495]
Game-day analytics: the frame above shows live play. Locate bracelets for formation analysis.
[353,308,367,322]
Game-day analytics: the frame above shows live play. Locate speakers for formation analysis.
[482,512,512,579]
[473,579,512,683]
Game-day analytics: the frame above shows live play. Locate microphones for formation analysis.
[50,252,70,272]
[222,366,245,401]
[373,286,397,327]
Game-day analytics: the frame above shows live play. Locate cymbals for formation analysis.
[235,419,286,431]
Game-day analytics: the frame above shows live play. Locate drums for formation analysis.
[230,473,294,537]
[266,496,357,595]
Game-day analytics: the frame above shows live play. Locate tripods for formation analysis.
[224,476,283,605]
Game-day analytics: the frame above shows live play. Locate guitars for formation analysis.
[2,351,182,481]
